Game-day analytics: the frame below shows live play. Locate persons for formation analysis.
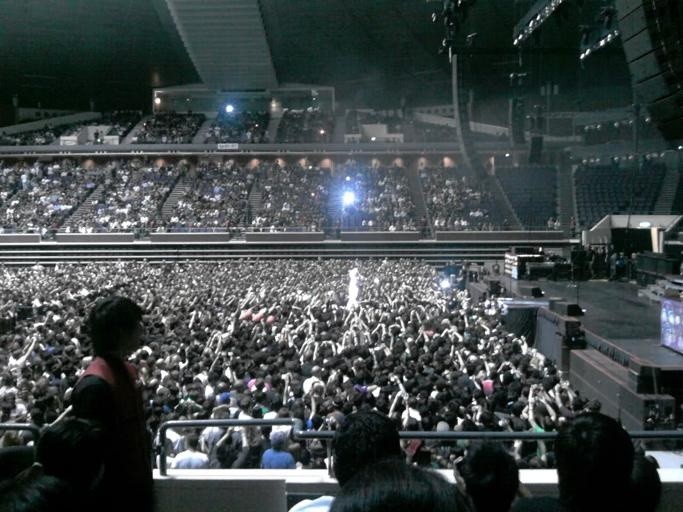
[1,112,683,511]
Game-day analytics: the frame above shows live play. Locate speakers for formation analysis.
[467,90,480,121]
[549,297,584,316]
[617,0,683,146]
[509,99,525,145]
[528,135,543,163]
[519,285,545,297]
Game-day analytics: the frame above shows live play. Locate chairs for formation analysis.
[492,160,668,233]
